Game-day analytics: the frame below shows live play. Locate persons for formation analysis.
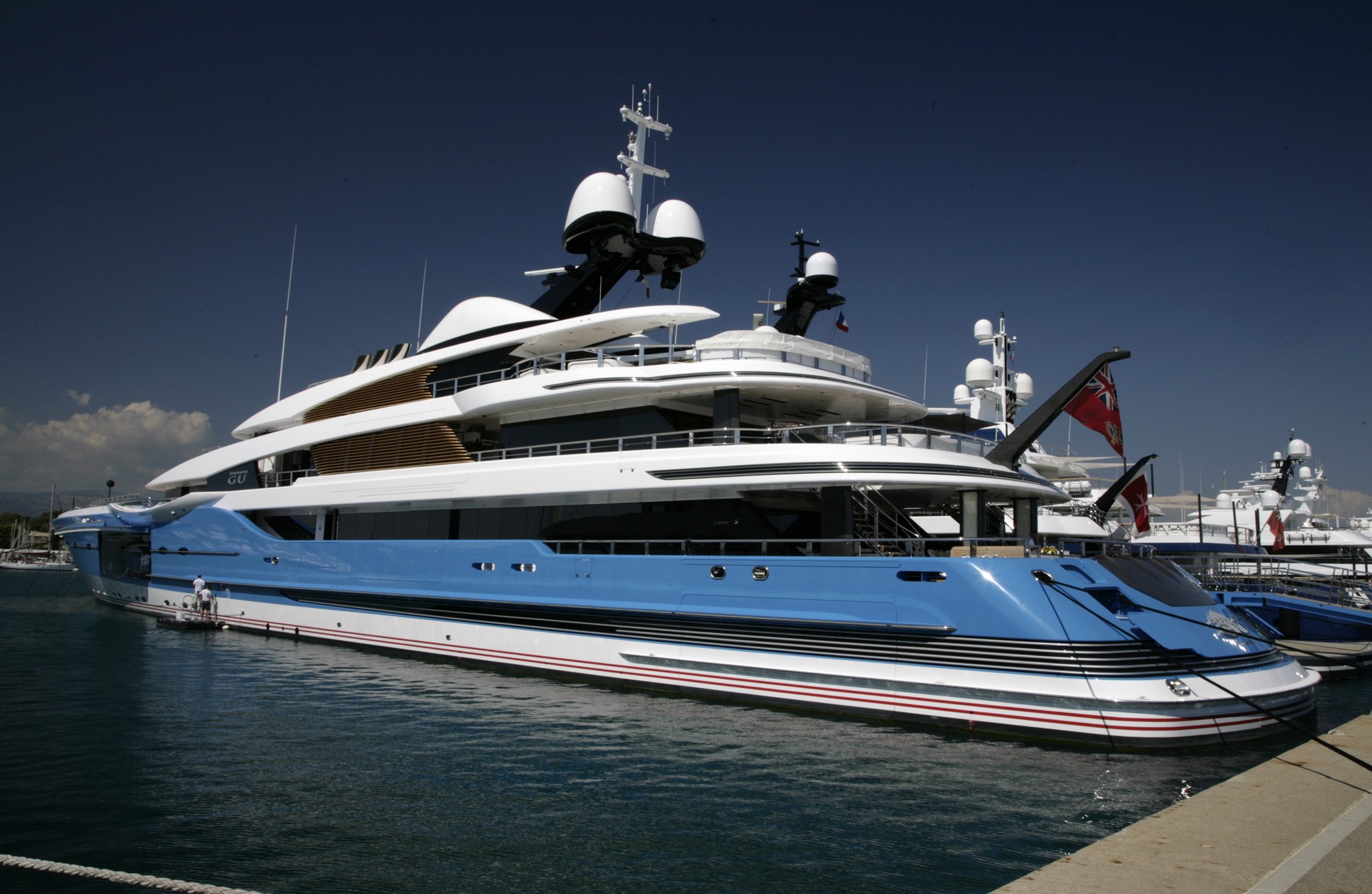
[221,585,230,598]
[193,575,205,611]
[200,586,212,620]
[140,551,151,573]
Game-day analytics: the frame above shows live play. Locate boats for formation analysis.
[0,517,79,573]
[945,307,1372,686]
[49,81,1326,757]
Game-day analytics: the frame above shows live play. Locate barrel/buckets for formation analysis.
[176,612,182,619]
[178,613,185,620]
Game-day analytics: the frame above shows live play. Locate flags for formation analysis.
[1267,503,1285,551]
[1114,464,1151,540]
[1064,362,1123,459]
[836,311,849,332]
[635,273,650,299]
